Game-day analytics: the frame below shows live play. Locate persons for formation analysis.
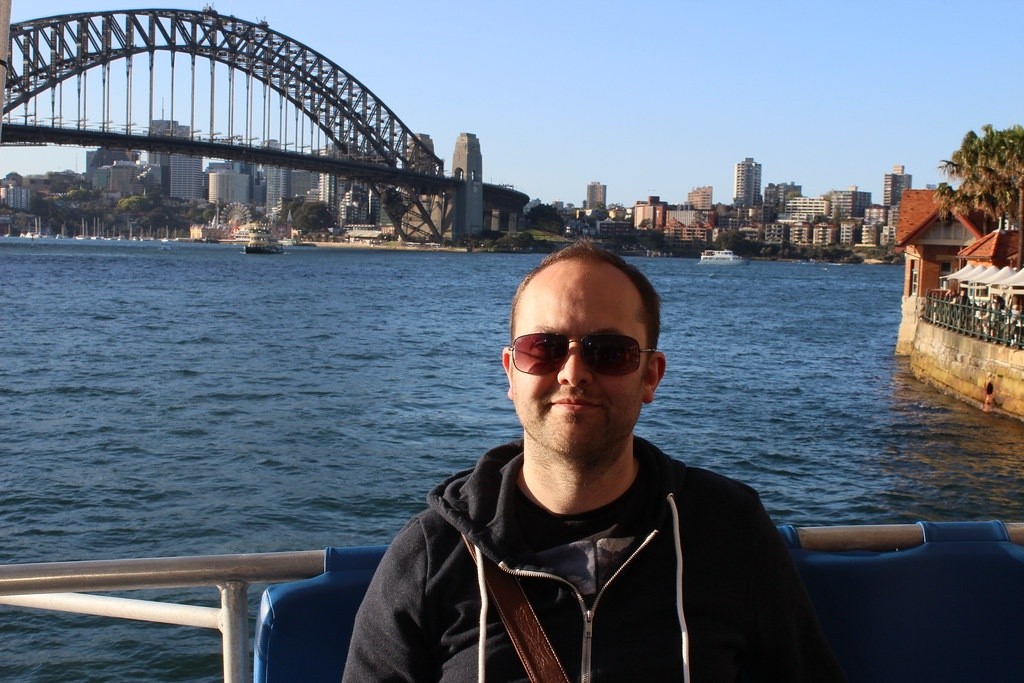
[342,237,836,683]
[931,289,1023,349]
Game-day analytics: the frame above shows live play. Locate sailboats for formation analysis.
[19,216,172,242]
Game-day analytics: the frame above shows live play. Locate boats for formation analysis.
[700,249,740,264]
[245,227,285,254]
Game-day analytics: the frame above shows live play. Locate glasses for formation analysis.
[508,332,656,377]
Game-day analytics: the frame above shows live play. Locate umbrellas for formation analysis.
[944,263,1024,310]
